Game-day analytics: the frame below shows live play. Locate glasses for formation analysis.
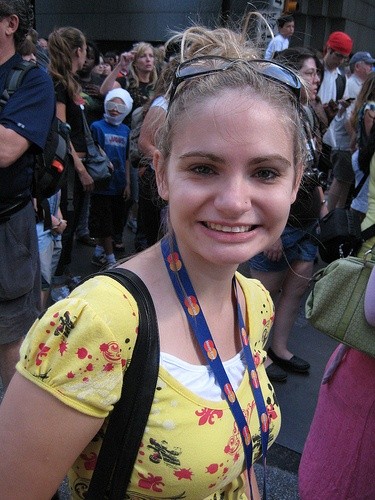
[163,55,301,119]
[106,102,128,113]
[332,51,348,60]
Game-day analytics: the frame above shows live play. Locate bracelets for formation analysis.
[322,200,327,205]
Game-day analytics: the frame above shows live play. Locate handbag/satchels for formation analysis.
[305,245,375,356]
[87,154,111,178]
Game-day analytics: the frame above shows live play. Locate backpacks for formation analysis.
[2,60,71,198]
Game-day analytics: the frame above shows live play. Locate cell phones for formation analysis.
[346,98,355,102]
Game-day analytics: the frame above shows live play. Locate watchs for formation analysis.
[51,221,62,230]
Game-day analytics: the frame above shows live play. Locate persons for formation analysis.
[0,23,314,500]
[0,0,375,386]
[298,261,375,500]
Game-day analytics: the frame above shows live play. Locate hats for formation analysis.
[327,32,352,56]
[350,51,375,67]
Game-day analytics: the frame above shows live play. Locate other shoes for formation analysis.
[268,347,311,372]
[264,362,290,381]
[91,254,114,269]
[127,218,138,234]
[52,285,70,302]
[80,233,94,246]
[67,277,82,287]
